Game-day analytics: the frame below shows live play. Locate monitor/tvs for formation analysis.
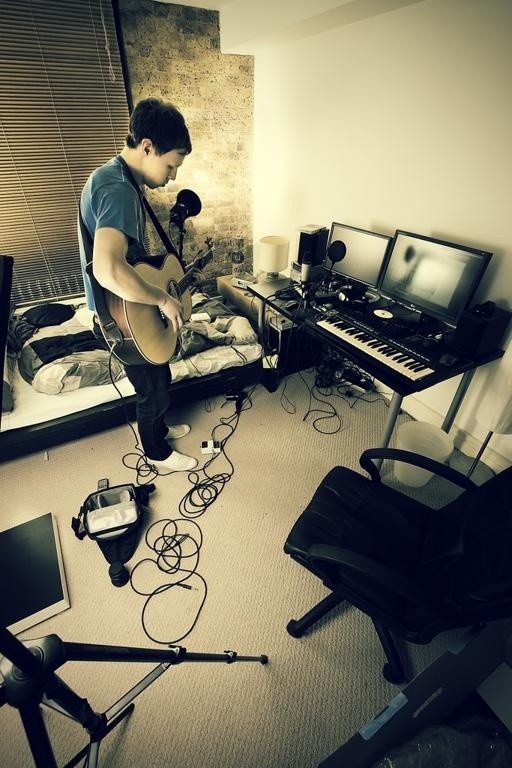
[377,228,494,326]
[322,222,392,304]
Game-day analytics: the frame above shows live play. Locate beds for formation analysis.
[0,292,264,463]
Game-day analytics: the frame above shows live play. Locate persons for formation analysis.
[77,98,198,470]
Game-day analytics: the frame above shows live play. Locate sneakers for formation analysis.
[145,450,198,472]
[166,424,191,440]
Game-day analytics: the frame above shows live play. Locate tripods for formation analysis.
[1,628,271,766]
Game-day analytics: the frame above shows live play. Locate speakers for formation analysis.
[452,303,512,364]
[297,229,328,268]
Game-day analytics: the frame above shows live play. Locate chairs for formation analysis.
[283,448,511,683]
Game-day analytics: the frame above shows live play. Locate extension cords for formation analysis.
[334,369,375,394]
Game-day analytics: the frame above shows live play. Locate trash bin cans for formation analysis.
[394,421,454,487]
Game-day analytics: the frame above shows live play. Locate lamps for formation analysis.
[259,236,290,282]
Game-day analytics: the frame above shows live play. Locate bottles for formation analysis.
[231,248,244,277]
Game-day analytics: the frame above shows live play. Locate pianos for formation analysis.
[306,305,439,397]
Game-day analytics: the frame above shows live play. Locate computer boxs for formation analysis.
[268,322,330,376]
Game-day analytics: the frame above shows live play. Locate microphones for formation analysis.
[300,251,313,293]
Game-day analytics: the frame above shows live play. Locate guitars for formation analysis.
[94,246,216,368]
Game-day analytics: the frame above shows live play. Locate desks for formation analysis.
[248,278,512,481]
